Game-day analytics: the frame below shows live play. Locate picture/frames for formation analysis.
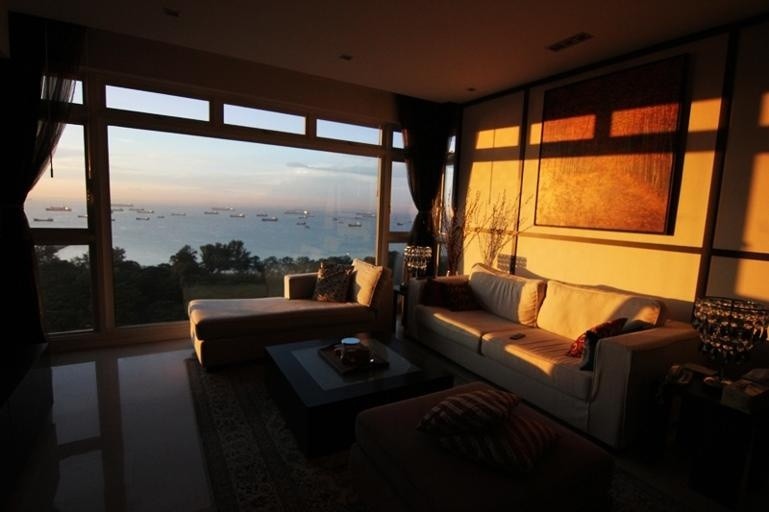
[534,53,690,234]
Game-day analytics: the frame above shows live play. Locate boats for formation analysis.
[288,209,310,228]
[334,210,374,228]
[204,206,280,222]
[396,219,413,226]
[35,204,184,222]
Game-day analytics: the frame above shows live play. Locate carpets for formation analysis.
[187,335,468,512]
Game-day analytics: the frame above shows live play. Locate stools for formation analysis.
[353,380,615,511]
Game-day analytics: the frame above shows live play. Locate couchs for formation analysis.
[408,272,700,458]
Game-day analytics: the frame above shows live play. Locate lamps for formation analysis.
[405,245,432,276]
[690,294,769,385]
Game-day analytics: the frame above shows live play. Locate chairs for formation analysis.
[187,267,397,372]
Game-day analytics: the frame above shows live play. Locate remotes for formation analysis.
[511,333,524,339]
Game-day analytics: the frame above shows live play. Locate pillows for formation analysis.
[436,413,575,474]
[312,261,354,303]
[427,265,662,370]
[415,389,519,450]
[351,258,384,307]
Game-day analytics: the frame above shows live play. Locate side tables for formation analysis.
[651,362,768,511]
[393,284,410,318]
